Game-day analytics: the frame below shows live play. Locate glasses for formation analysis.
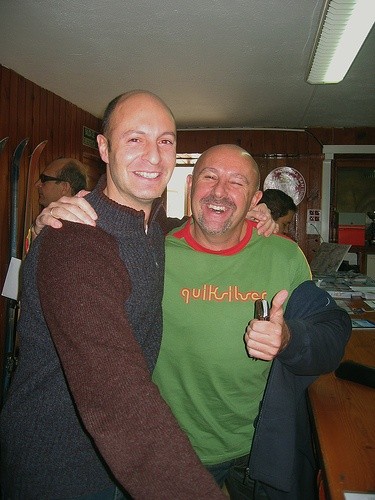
[39,173,67,183]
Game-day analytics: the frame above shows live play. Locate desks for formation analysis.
[307,273,375,500]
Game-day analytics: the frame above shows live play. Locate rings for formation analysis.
[50,207,57,219]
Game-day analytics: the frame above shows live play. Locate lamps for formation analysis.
[306,0,375,84]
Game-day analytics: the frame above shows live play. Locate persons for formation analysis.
[35,157,86,206]
[1,87,283,498]
[257,189,299,235]
[28,143,352,499]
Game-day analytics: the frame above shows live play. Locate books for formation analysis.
[313,268,375,329]
